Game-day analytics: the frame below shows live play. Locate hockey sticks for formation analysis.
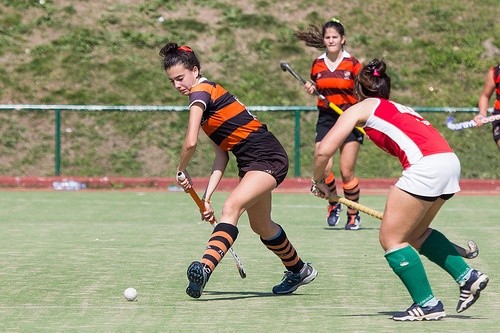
[309,185,479,260]
[280,62,367,136]
[177,171,247,279]
[446,115,500,131]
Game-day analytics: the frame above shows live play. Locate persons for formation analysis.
[159,42,318,298]
[472,64,500,148]
[312,59,489,322]
[294,18,365,230]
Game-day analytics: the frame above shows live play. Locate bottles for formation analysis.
[52,182,87,191]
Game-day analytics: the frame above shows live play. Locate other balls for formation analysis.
[124,288,137,301]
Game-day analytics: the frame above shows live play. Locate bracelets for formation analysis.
[202,197,211,203]
[312,178,325,184]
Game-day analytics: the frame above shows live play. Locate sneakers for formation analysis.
[272,262,318,294]
[456,269,489,313]
[185,261,208,298]
[327,200,342,226]
[345,209,360,229]
[393,299,446,321]
